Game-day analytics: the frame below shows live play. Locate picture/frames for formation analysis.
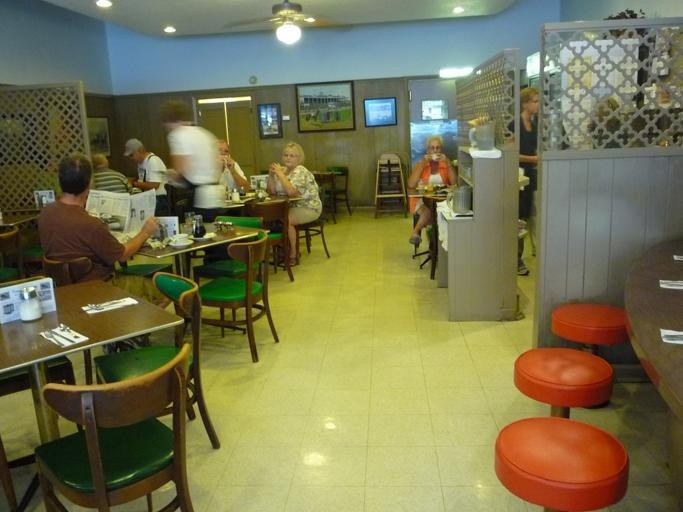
[294,80,356,133]
[256,103,284,140]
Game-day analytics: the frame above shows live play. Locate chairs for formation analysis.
[91,270,224,450]
[122,166,350,281]
[41,255,93,285]
[32,343,195,512]
[197,230,280,364]
[116,258,174,280]
[192,215,265,331]
[2,212,38,277]
[1,436,39,511]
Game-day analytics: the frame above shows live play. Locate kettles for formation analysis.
[191,214,206,238]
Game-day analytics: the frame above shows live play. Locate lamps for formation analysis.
[272,0,304,46]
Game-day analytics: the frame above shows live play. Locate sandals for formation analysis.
[288,252,301,266]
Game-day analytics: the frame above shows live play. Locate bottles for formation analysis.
[17,286,41,322]
[225,184,245,204]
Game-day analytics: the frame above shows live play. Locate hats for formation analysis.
[125,139,142,156]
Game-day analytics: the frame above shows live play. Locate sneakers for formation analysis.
[518,265,530,276]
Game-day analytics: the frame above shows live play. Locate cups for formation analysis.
[432,153,438,161]
[447,190,472,213]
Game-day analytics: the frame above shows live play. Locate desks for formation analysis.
[133,222,271,335]
[0,278,185,444]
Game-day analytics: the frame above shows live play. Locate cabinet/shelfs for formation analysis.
[436,146,504,322]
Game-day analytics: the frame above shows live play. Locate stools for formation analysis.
[494,303,630,512]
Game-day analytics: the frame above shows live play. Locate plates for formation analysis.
[188,232,216,242]
[169,239,193,249]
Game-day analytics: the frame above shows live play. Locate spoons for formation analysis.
[84,299,127,312]
[45,322,81,346]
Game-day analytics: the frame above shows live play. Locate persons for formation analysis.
[589,98,628,149]
[265,141,322,267]
[160,98,226,221]
[124,138,172,211]
[38,155,171,347]
[406,134,458,245]
[505,87,540,275]
[212,137,249,195]
[90,154,132,194]
